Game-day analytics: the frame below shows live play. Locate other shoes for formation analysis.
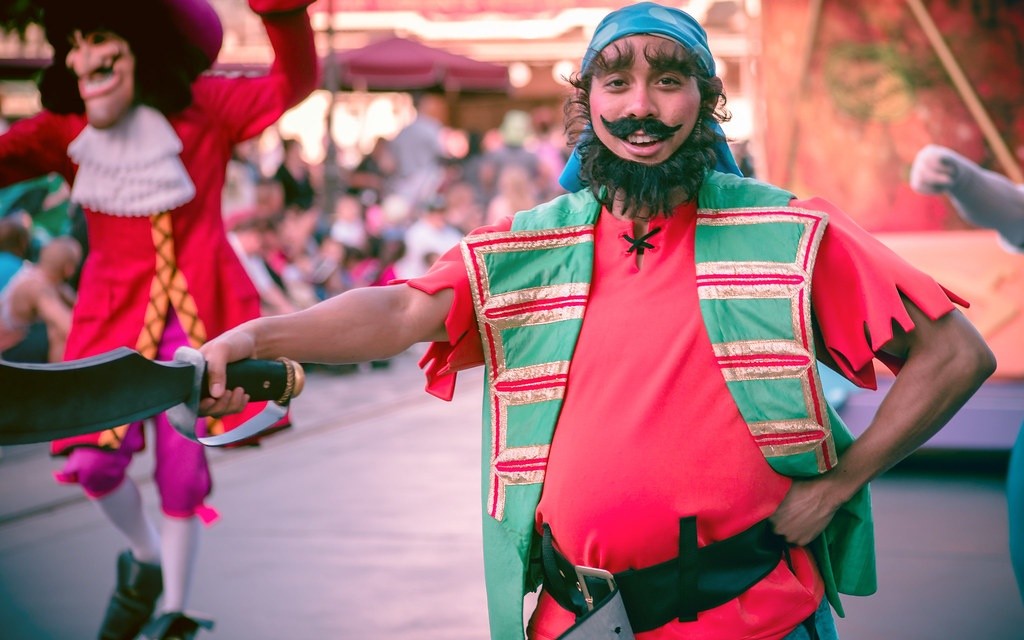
[132,608,214,640]
[97,548,163,640]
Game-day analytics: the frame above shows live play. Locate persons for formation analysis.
[0,211,86,362]
[0,0,318,640]
[222,91,575,375]
[196,1,997,640]
[909,145,1024,597]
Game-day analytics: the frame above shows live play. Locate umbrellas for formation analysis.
[318,34,508,94]
